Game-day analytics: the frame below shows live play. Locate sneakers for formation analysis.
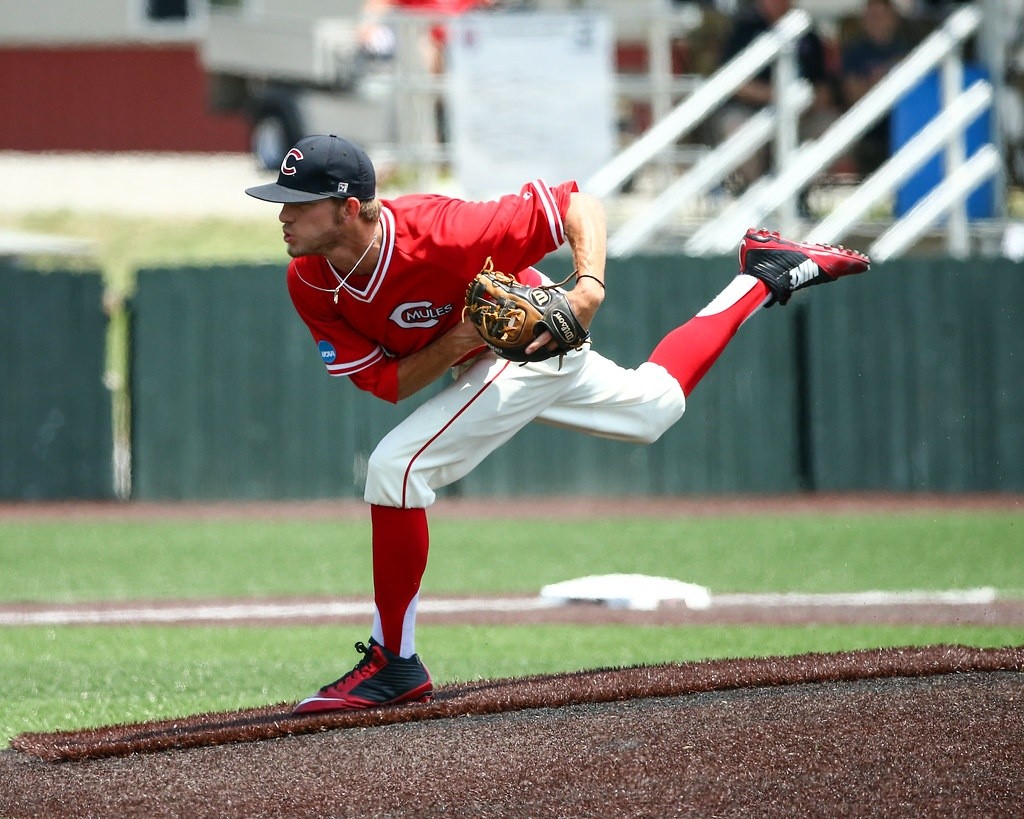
[292,641,437,718]
[738,226,872,309]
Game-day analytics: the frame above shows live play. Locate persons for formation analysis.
[359,0,974,218]
[244,134,872,714]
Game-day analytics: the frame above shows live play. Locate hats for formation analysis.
[244,134,377,204]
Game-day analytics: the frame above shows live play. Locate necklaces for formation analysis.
[292,221,381,302]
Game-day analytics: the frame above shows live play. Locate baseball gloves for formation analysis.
[464,269,591,363]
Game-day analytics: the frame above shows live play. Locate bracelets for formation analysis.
[576,275,605,289]
